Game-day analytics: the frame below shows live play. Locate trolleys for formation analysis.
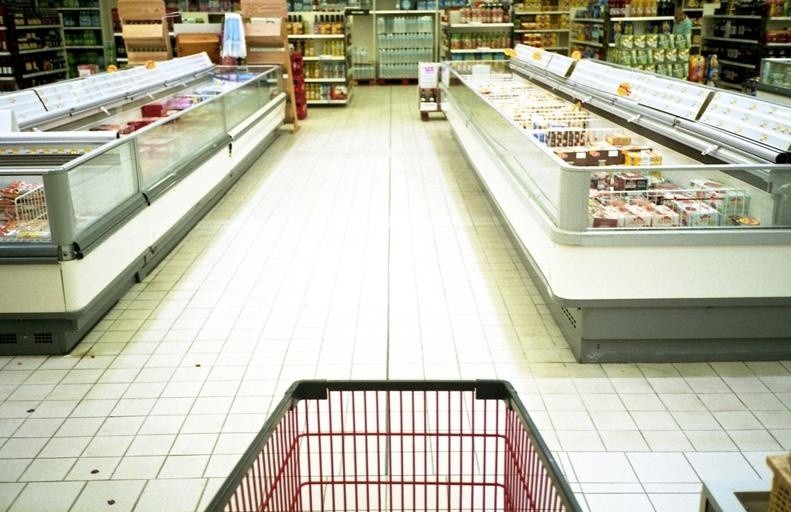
[192,376,583,512]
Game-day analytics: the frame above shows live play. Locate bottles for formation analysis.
[354,0,435,79]
[438,1,511,60]
[287,7,352,101]
[0,0,100,83]
[623,0,675,17]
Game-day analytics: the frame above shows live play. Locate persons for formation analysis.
[672,6,693,53]
